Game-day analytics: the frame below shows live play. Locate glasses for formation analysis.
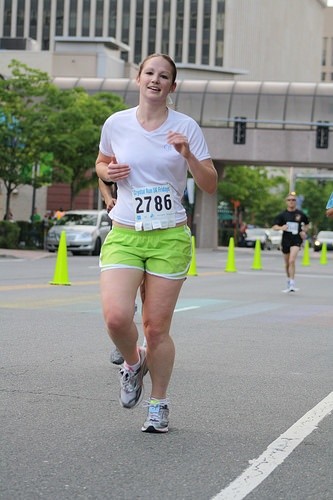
[286,199,296,201]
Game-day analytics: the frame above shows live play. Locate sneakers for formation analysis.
[143,337,147,347]
[120,347,148,408]
[287,280,295,292]
[110,349,124,364]
[141,398,169,432]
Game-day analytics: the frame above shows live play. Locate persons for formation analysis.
[325,193,333,218]
[95,54,217,434]
[3,207,65,245]
[98,155,146,364]
[271,194,310,292]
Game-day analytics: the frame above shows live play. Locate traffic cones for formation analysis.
[318,241,328,265]
[224,237,238,272]
[300,239,311,267]
[250,238,263,269]
[186,235,198,276]
[48,231,72,285]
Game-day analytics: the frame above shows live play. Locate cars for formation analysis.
[218,200,283,252]
[46,208,112,256]
[313,230,333,252]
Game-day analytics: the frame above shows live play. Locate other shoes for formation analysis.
[18,245,25,249]
[36,246,43,249]
